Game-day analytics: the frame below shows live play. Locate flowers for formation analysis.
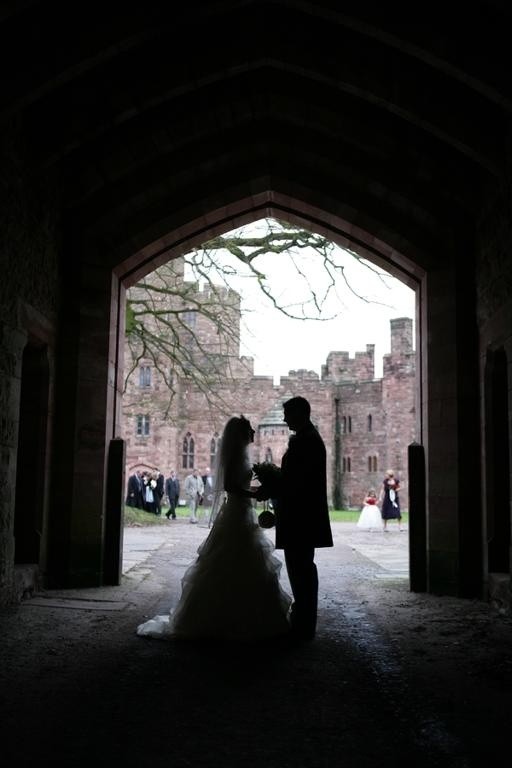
[252,462,282,502]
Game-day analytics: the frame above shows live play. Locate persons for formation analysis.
[363,486,384,533]
[377,468,406,533]
[208,415,273,645]
[126,466,216,524]
[254,396,334,645]
